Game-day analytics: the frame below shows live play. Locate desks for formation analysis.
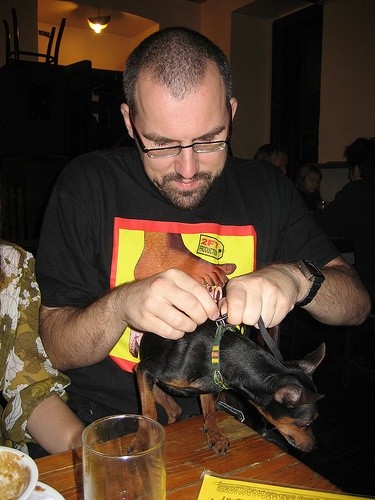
[31,409,343,500]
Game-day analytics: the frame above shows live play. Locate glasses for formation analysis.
[128,98,234,159]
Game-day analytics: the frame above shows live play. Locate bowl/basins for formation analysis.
[0,446,38,500]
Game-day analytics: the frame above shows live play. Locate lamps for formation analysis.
[87,5,112,34]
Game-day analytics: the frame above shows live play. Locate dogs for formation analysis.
[126,317,326,475]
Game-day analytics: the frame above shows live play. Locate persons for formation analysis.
[252,143,288,175]
[35,26,371,442]
[317,136,375,331]
[298,162,322,210]
[0,242,88,456]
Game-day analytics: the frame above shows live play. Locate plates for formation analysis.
[26,481,66,500]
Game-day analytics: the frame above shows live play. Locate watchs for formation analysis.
[296,260,325,308]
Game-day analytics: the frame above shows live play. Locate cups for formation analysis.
[82,414,168,500]
